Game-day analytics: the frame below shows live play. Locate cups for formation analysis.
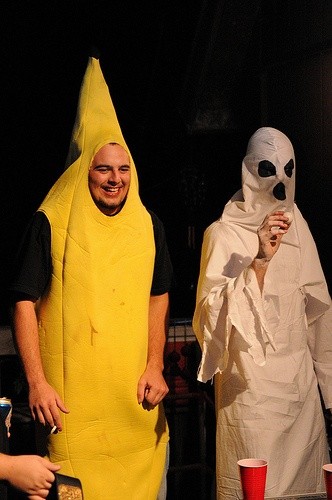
[237,458,269,500]
[322,464,332,500]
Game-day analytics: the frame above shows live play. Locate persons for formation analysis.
[192,127,332,500]
[0,453,62,500]
[164,344,200,432]
[0,55,169,500]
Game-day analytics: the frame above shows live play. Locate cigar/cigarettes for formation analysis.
[51,426,57,434]
